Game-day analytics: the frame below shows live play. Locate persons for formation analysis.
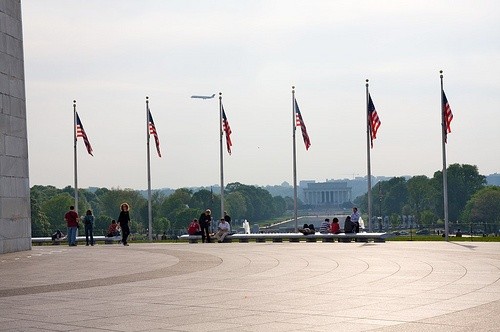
[352,207,360,233]
[118,203,130,246]
[50,226,63,245]
[224,212,232,235]
[199,209,214,243]
[303,224,315,234]
[82,210,95,246]
[330,218,341,234]
[344,216,355,233]
[106,220,121,237]
[65,205,80,246]
[320,219,333,234]
[188,218,200,235]
[456,229,462,237]
[215,218,231,243]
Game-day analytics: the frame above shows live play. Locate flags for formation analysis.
[222,107,232,156]
[442,91,453,144]
[368,94,381,150]
[147,110,161,157]
[75,112,93,156]
[294,99,311,151]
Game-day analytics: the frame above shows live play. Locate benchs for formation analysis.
[178,231,388,243]
[31,237,121,243]
